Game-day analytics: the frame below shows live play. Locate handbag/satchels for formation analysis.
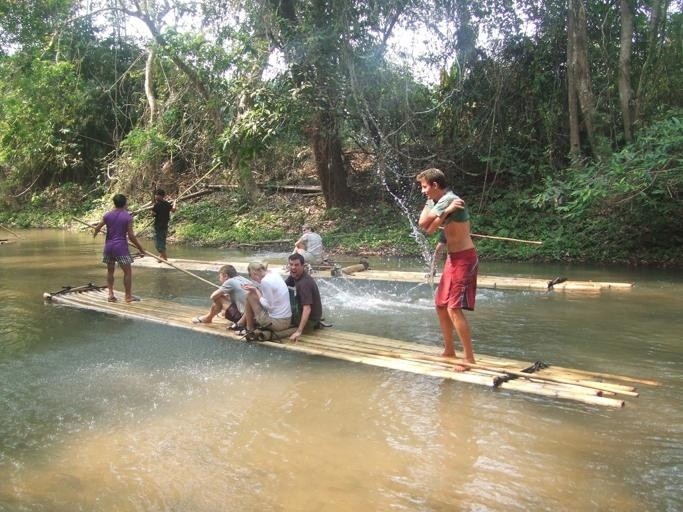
[288,288,298,325]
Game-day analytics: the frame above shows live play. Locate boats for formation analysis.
[128,252,638,298]
[39,283,662,416]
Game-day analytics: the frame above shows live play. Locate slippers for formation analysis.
[191,315,212,323]
[125,296,140,302]
[107,297,117,302]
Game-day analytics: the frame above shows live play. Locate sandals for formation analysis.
[226,322,250,335]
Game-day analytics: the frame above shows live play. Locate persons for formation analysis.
[283,254,322,342]
[416,168,479,372]
[94,193,144,303]
[152,189,178,263]
[291,223,322,266]
[226,261,292,335]
[192,264,253,325]
[425,223,446,278]
